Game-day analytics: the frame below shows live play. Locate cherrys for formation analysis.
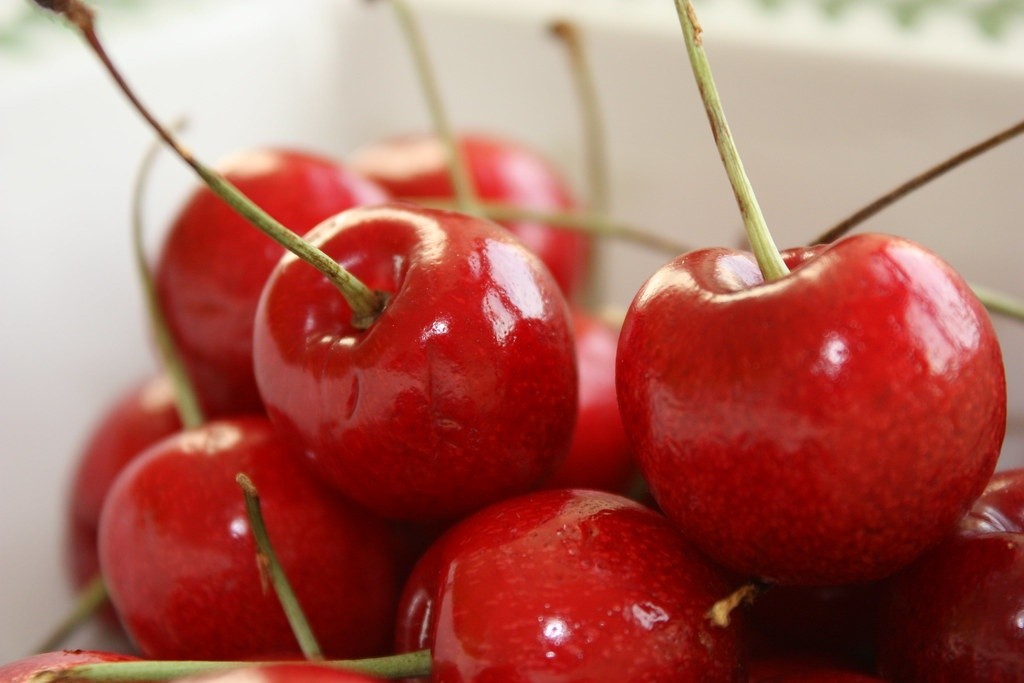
[33,0,1024,683]
